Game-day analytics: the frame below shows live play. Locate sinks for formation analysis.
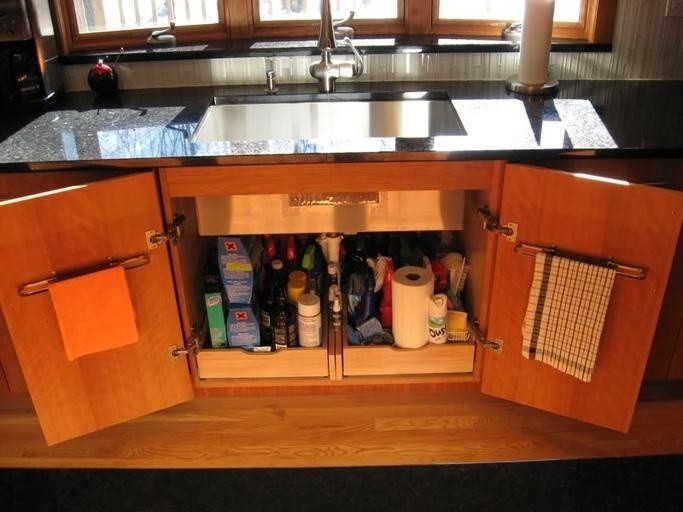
[191,90,465,141]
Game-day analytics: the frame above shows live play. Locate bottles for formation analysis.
[429,293,450,345]
[327,287,335,327]
[269,258,299,351]
[297,293,324,347]
[286,279,307,306]
[357,278,376,324]
[288,270,307,282]
[258,264,274,350]
[332,297,342,333]
[378,262,396,328]
[325,265,339,287]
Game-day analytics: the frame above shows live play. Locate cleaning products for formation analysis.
[429,296,447,344]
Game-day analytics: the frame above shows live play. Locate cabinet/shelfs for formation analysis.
[0,158,683,468]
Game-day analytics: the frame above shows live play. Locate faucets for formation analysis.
[310,0,364,91]
